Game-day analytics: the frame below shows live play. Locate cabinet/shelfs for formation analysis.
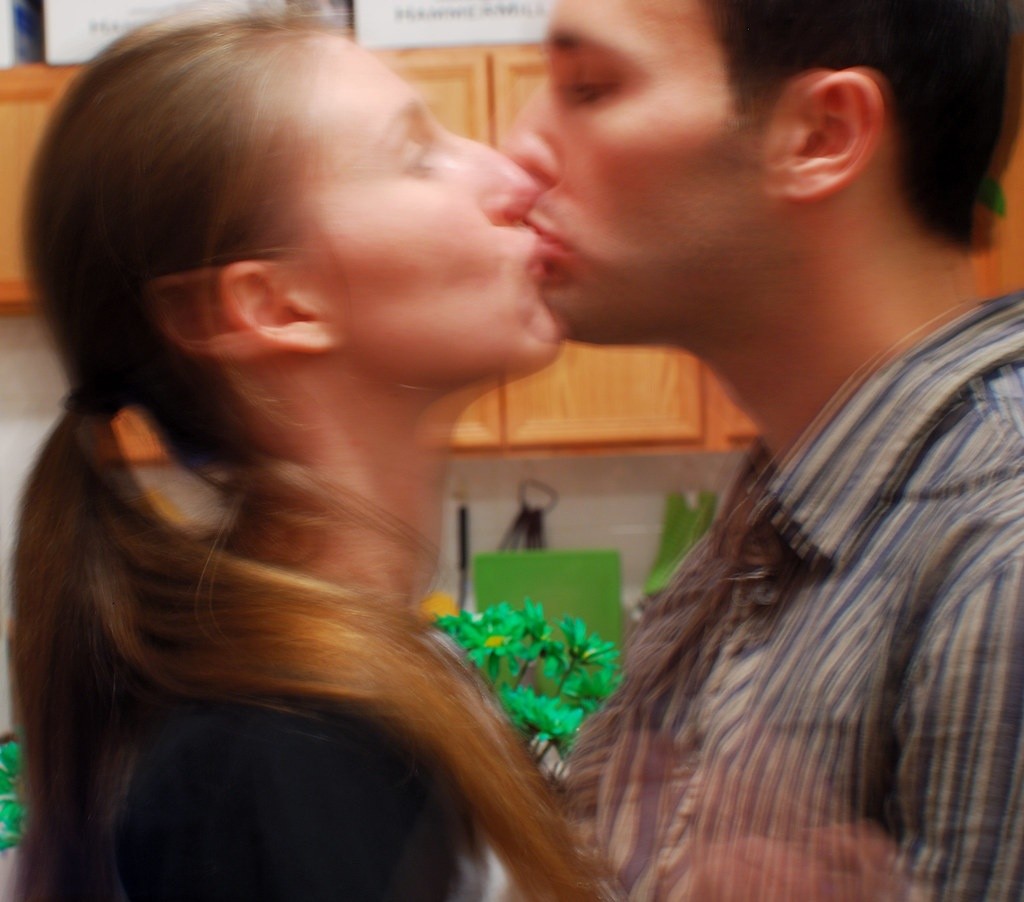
[0,44,1023,471]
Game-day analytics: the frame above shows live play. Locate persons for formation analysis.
[9,16,623,902]
[500,0,1024,902]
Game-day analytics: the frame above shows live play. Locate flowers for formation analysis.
[436,597,622,765]
